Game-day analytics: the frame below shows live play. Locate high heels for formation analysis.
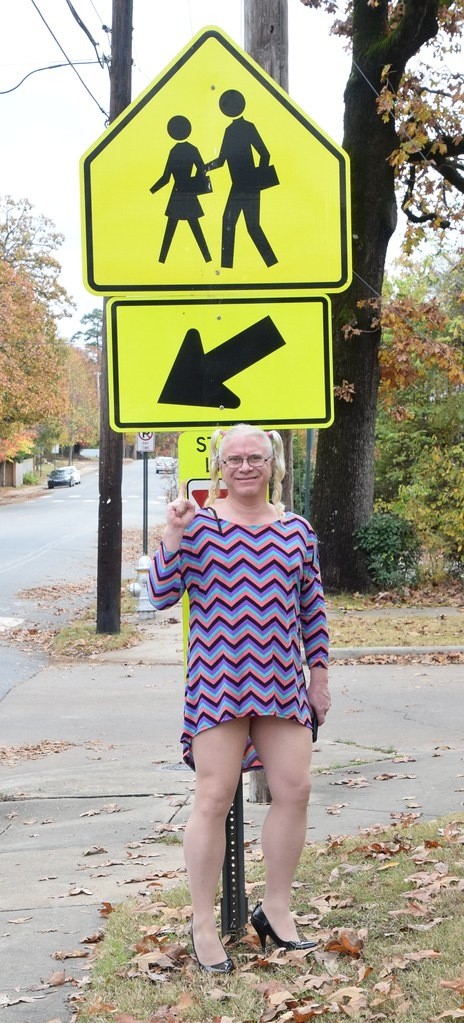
[190,914,235,973]
[251,904,317,952]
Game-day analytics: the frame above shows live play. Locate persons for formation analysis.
[148,425,331,973]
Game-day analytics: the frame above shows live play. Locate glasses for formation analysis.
[220,455,271,469]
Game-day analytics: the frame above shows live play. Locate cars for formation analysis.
[155,456,177,473]
[46,465,81,489]
